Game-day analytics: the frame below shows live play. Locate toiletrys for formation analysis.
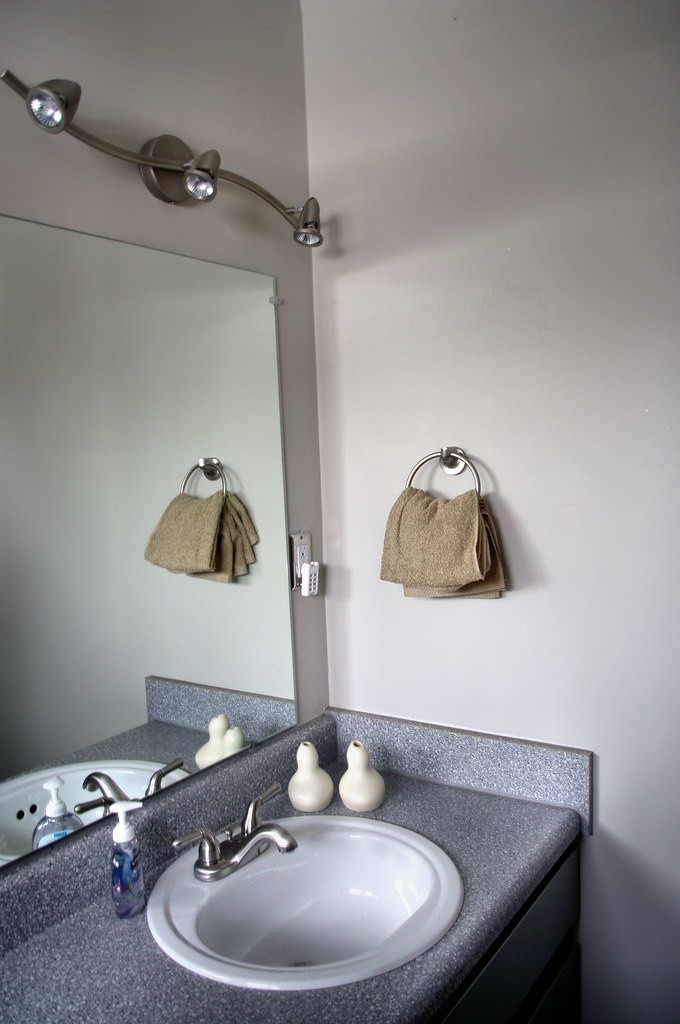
[32,776,84,852]
[107,800,147,919]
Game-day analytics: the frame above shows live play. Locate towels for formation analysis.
[379,486,509,601]
[142,488,260,583]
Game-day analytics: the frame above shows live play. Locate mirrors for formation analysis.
[0,213,299,868]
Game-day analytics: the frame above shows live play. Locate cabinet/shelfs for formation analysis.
[439,832,582,1024]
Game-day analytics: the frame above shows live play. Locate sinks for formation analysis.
[147,816,464,991]
[0,758,191,867]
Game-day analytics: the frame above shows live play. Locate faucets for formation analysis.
[170,781,299,882]
[73,756,184,814]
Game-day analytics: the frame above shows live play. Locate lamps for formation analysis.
[1,69,323,247]
